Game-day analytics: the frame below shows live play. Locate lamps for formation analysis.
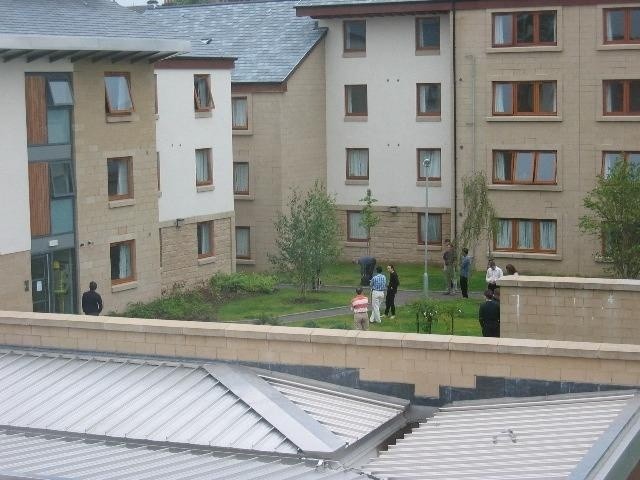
[175,218,186,228]
[388,206,397,215]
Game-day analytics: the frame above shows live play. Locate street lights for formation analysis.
[421,159,433,293]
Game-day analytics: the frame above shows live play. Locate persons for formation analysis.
[350,287,370,331]
[458,248,470,300]
[351,255,376,286]
[82,281,102,316]
[443,238,458,291]
[369,266,387,323]
[442,243,457,296]
[380,264,400,319]
[479,260,519,338]
